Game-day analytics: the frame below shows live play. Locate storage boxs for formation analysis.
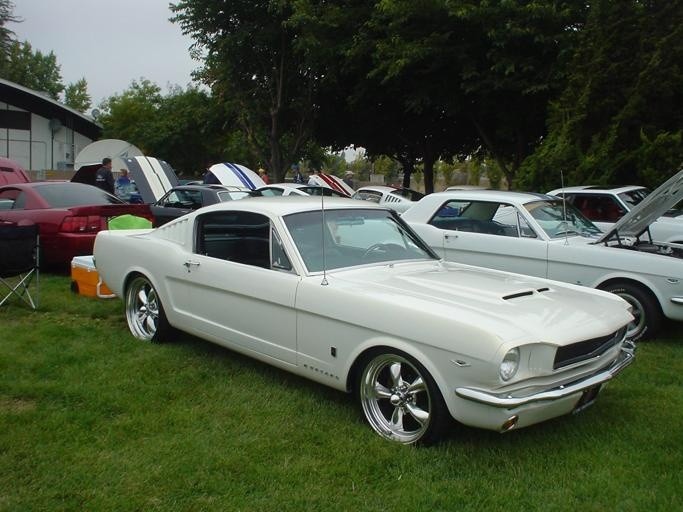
[69,253,114,299]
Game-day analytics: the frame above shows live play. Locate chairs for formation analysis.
[0,219,44,312]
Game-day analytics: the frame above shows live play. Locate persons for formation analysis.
[342,170,354,187]
[114,168,130,197]
[95,157,114,192]
[290,164,304,184]
[204,169,217,184]
[258,168,270,184]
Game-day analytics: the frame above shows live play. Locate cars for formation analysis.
[330,168,683,347]
[542,180,683,257]
[1,153,157,276]
[89,191,645,454]
